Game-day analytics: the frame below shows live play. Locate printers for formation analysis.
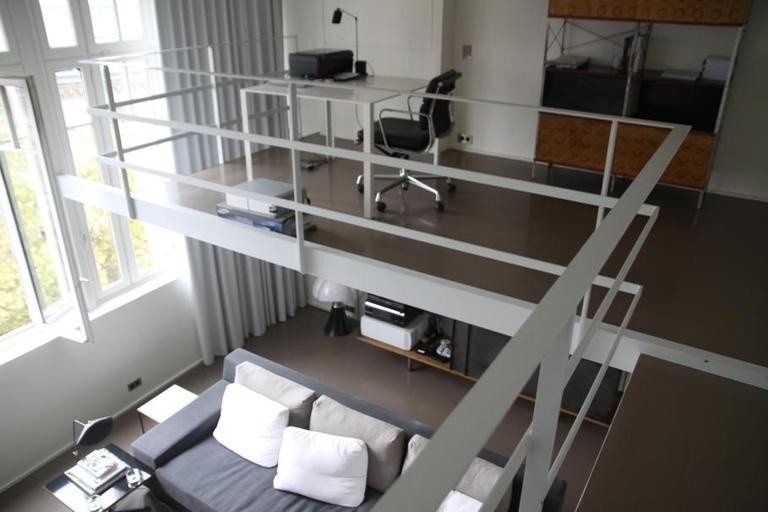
[288,47,361,82]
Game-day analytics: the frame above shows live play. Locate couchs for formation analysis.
[131,347,566,512]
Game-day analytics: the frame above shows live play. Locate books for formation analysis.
[69,447,128,490]
[64,447,132,496]
[77,448,118,478]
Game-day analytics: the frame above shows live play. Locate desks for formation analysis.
[239,70,433,220]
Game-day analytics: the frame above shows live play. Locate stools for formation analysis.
[137,383,201,434]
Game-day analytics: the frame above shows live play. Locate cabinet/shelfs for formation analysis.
[532,0,749,216]
[40,442,156,512]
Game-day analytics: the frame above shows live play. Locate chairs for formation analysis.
[357,68,464,213]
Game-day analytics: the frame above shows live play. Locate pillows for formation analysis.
[212,382,486,512]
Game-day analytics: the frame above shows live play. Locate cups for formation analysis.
[86,494,103,512]
[354,60,367,78]
[125,467,141,484]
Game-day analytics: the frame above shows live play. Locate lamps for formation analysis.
[313,277,355,337]
[71,415,111,478]
[330,7,372,82]
